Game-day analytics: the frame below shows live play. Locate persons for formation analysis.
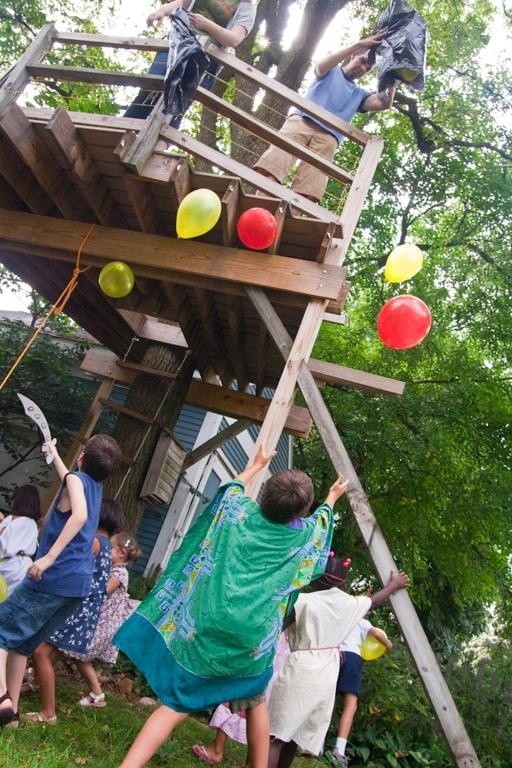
[119,0,257,133]
[251,26,398,204]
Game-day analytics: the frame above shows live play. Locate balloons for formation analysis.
[376,294,432,352]
[235,207,278,252]
[98,261,136,299]
[384,243,425,284]
[175,188,223,240]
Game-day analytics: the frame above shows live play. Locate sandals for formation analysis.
[0,688,20,730]
[25,711,58,726]
[20,681,42,696]
[77,691,107,708]
[192,744,218,765]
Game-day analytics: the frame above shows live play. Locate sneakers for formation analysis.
[324,746,348,768]
